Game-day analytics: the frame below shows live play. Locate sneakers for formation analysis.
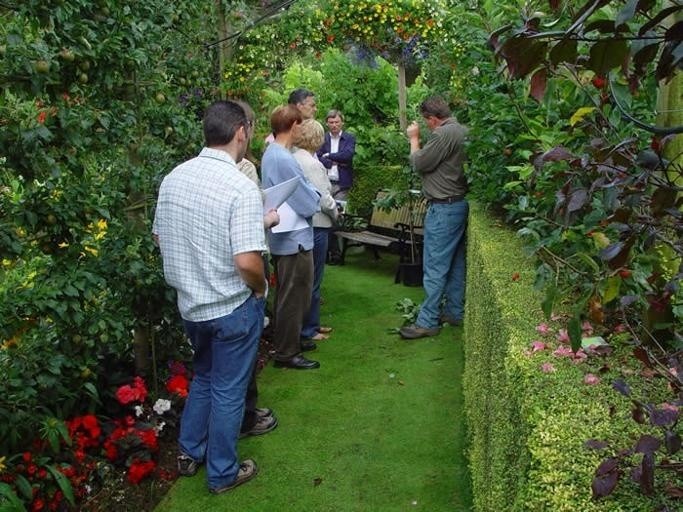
[399,322,440,338]
[176,455,205,477]
[255,408,273,416]
[439,313,462,326]
[239,416,277,439]
[208,458,259,494]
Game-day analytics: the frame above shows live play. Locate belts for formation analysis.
[433,195,462,204]
[330,180,339,184]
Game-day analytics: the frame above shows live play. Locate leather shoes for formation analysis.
[273,324,333,370]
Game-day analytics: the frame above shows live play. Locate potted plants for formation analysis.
[369,127,428,287]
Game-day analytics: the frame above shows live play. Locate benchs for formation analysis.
[334,191,427,283]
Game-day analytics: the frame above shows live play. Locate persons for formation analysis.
[230,99,277,437]
[290,119,338,342]
[150,101,268,495]
[398,94,468,340]
[288,87,318,119]
[261,105,320,371]
[318,109,356,263]
